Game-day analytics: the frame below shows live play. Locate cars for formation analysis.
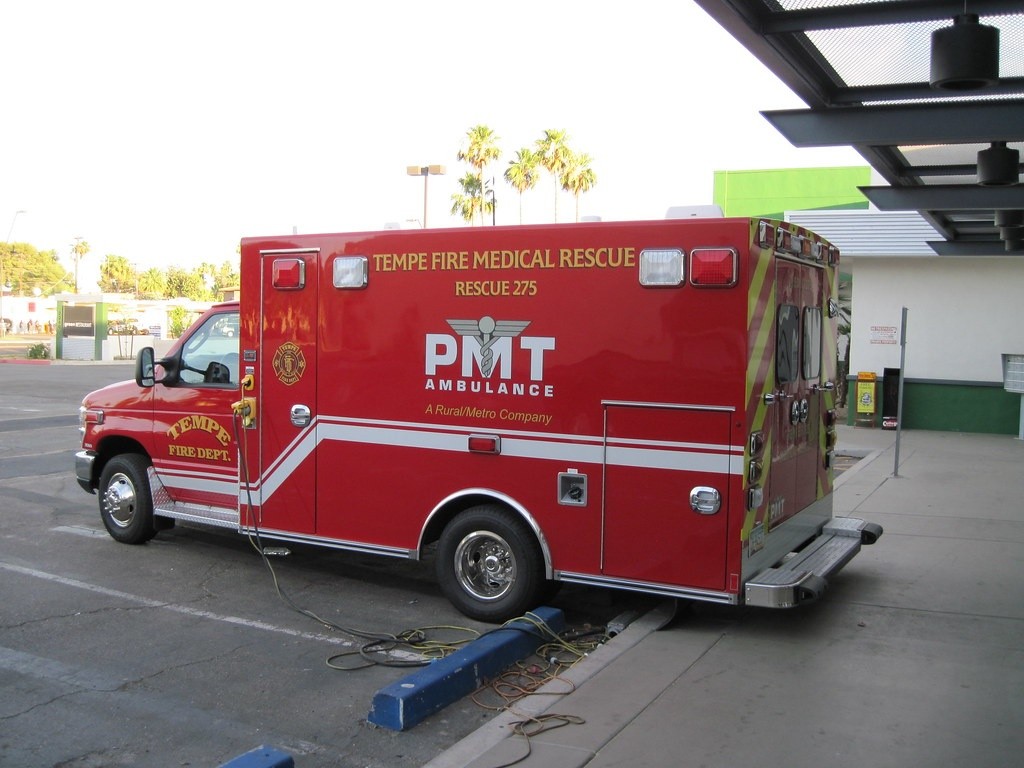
[107,325,125,335]
[221,322,240,338]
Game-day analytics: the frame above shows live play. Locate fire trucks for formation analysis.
[70,215,886,625]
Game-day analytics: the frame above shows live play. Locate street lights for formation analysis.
[405,164,445,228]
